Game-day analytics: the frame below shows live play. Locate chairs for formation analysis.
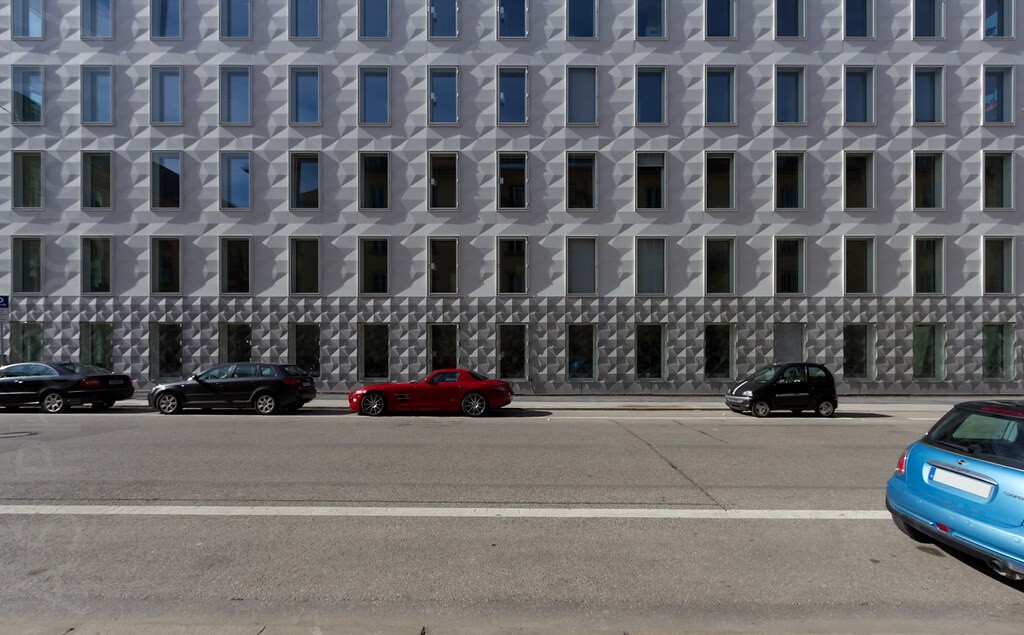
[787,369,798,378]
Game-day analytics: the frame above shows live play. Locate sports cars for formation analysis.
[347,368,514,418]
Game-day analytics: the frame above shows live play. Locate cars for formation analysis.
[144,361,318,416]
[0,360,135,414]
[884,398,1024,587]
[724,361,839,419]
